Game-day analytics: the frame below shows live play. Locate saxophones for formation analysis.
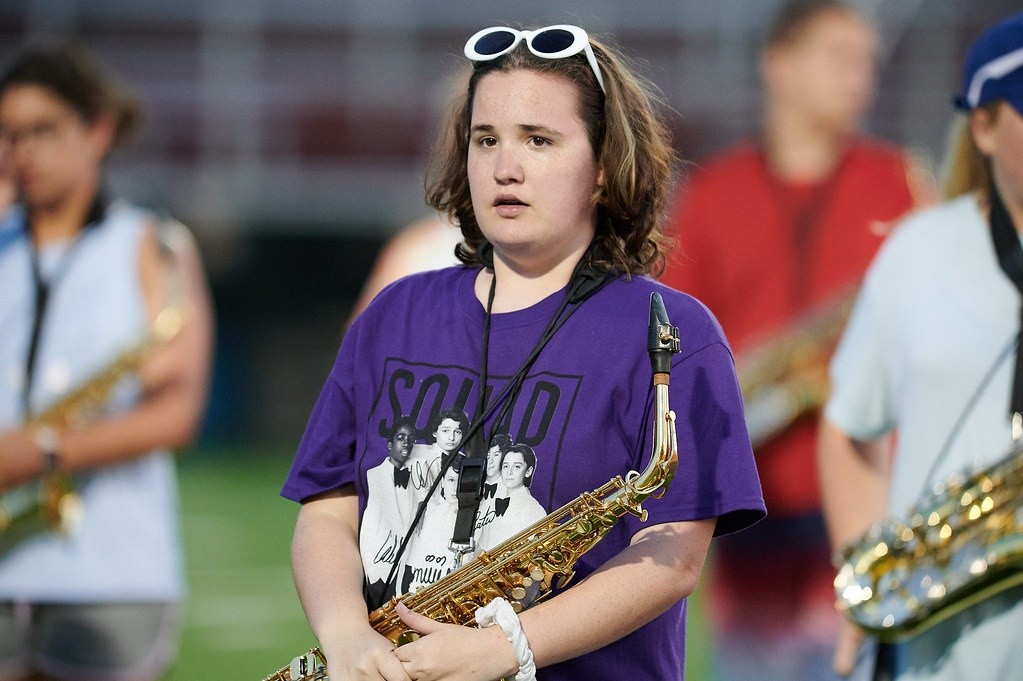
[260,286,683,681]
[1,290,195,557]
[831,440,1023,644]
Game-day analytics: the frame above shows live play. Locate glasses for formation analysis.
[464,25,606,99]
[0,101,73,151]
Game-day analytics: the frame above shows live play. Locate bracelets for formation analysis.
[474,597,537,680]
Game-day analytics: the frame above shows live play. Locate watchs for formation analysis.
[28,422,62,476]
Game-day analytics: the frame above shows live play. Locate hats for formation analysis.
[951,9,1023,119]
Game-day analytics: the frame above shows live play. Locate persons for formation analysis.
[280,24,767,681]
[0,57,215,681]
[345,0,1023,681]
